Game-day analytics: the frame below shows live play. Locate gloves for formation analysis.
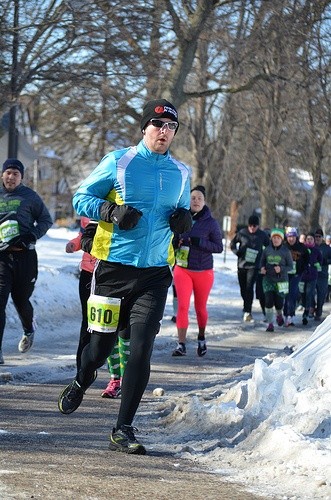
[233,249,242,257]
[7,232,33,248]
[100,200,143,231]
[169,207,193,235]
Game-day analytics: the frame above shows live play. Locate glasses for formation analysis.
[150,119,178,131]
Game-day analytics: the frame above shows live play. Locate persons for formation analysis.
[0,159,53,363]
[258,228,293,332]
[58,99,192,454]
[66,216,98,373]
[172,185,223,356]
[257,226,331,327]
[102,306,131,399]
[230,215,270,321]
[171,268,177,322]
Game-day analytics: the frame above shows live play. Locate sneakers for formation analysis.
[57,368,97,414]
[108,423,146,454]
[100,378,122,398]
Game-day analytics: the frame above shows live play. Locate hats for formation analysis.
[316,229,324,236]
[1,158,24,180]
[249,215,259,225]
[271,227,285,240]
[140,98,180,136]
[286,226,299,238]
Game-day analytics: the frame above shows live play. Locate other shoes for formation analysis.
[172,345,187,355]
[197,339,208,356]
[17,331,34,352]
[309,306,316,317]
[243,311,252,322]
[285,320,295,328]
[266,323,275,333]
[315,316,321,321]
[277,314,285,327]
[0,351,4,364]
[302,317,308,325]
